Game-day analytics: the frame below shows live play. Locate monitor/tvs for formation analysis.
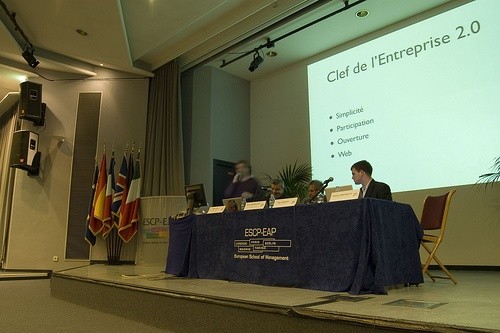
[184,182,208,215]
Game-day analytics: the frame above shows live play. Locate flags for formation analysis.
[85,150,143,246]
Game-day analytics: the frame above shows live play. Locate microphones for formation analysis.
[323,177,333,184]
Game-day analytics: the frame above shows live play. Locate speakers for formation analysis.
[18,81,42,119]
[7,130,39,168]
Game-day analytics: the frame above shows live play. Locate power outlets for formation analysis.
[53,256,59,261]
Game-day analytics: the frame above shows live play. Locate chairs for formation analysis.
[410,188,456,288]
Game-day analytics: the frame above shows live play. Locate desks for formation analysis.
[164,198,425,296]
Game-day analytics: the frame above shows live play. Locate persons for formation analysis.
[350,160,392,201]
[264,178,291,200]
[224,160,265,202]
[304,180,327,204]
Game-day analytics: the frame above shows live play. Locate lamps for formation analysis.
[22,48,40,69]
[248,48,263,72]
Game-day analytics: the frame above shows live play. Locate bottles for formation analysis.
[269,194,275,208]
[317,191,324,204]
[240,197,246,211]
